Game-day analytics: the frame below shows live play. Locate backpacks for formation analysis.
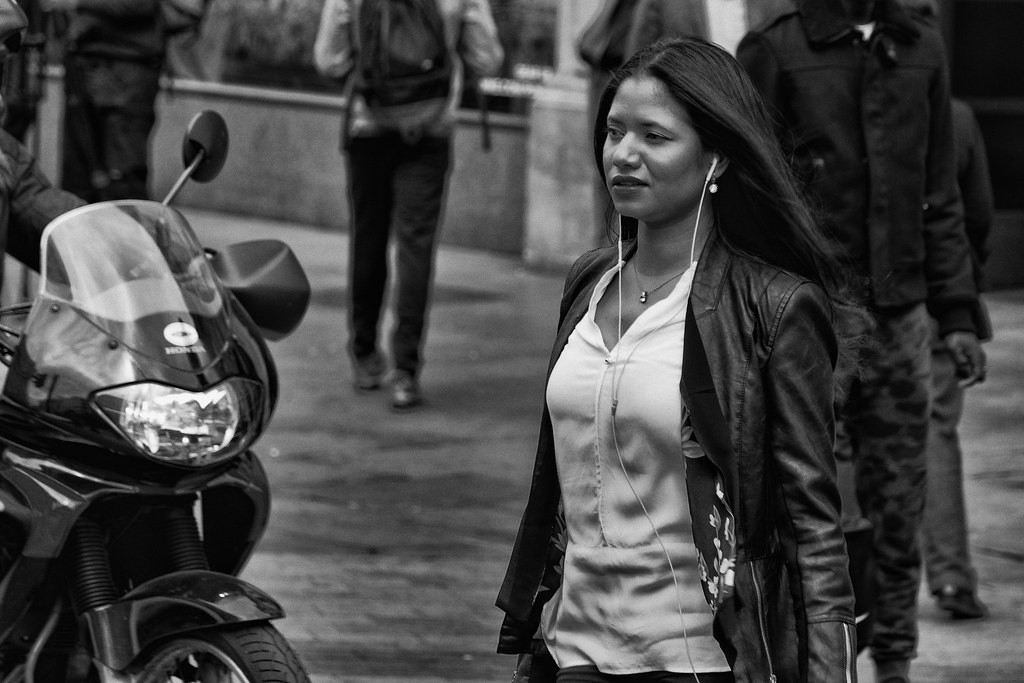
[341,0,465,137]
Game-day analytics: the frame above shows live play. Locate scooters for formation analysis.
[0,108,319,682]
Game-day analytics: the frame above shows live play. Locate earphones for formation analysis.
[704,152,721,181]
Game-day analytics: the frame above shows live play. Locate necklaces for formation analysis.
[634,250,691,303]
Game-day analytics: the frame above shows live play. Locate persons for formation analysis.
[495,32,856,683]
[0,0,213,292]
[578,0,993,683]
[314,0,504,406]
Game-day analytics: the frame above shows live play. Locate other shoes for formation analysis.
[354,353,378,389]
[393,367,415,408]
[939,582,984,615]
